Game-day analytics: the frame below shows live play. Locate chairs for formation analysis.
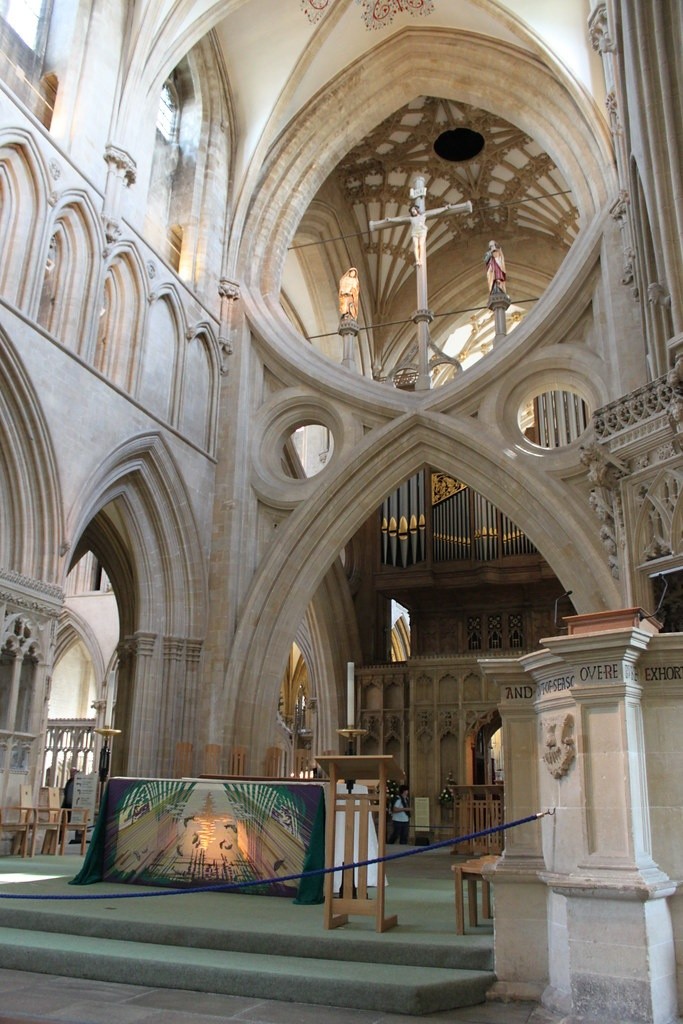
[0,785,90,858]
[449,854,501,934]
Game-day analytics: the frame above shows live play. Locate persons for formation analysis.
[387,785,414,845]
[646,283,672,306]
[385,203,453,266]
[338,267,359,322]
[58,767,82,845]
[672,407,683,432]
[483,240,507,295]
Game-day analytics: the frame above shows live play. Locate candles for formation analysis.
[346,662,355,725]
[105,671,116,726]
